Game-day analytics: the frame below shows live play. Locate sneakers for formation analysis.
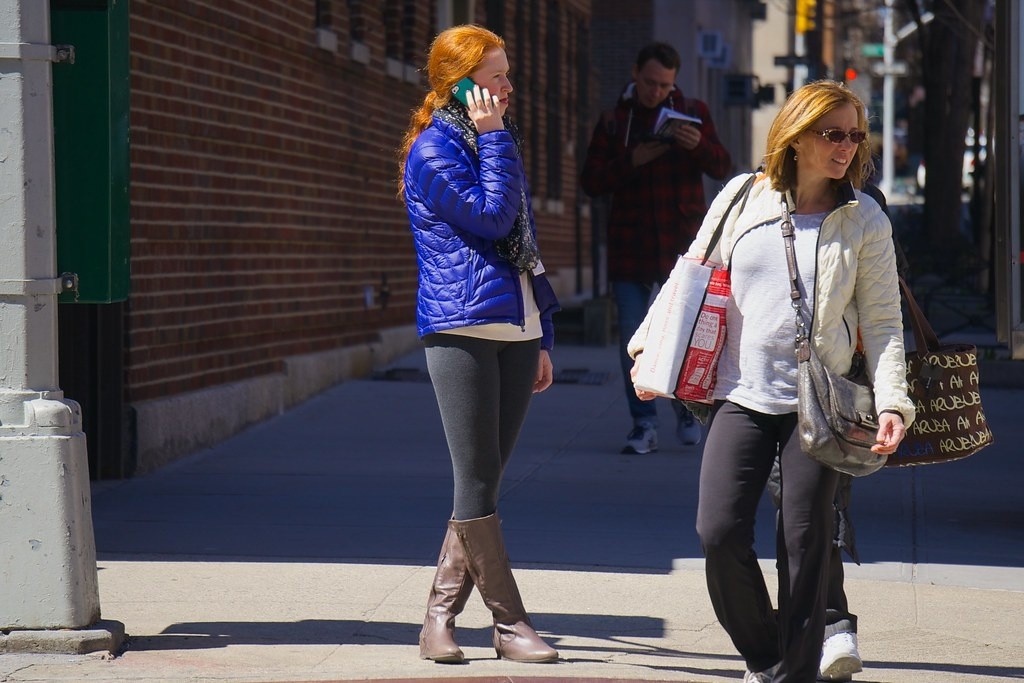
[622,425,658,453]
[742,659,789,683]
[671,401,702,445]
[815,632,863,683]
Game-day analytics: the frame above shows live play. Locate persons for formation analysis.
[580,43,731,454]
[398,24,558,663]
[626,81,916,683]
[755,165,911,681]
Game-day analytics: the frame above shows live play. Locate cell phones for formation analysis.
[451,77,493,108]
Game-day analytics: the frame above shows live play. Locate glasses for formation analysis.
[806,128,865,144]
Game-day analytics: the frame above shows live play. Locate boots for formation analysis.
[448,509,560,662]
[419,509,474,663]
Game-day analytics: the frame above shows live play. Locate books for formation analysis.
[654,108,702,139]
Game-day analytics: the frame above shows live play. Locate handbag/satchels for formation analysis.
[632,172,758,403]
[794,333,889,478]
[881,277,993,468]
